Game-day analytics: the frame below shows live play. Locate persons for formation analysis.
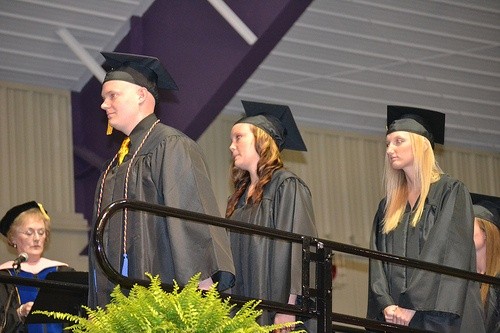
[364,104,488,333]
[0,200,80,333]
[88,49,235,315]
[223,99,320,333]
[465,192,500,333]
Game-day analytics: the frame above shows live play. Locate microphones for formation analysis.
[13,253,28,265]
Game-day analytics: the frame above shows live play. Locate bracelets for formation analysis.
[19,304,25,317]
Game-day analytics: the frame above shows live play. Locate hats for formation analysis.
[468,192,500,230]
[0,201,49,238]
[234,100,308,152]
[100,51,180,135]
[385,105,445,150]
